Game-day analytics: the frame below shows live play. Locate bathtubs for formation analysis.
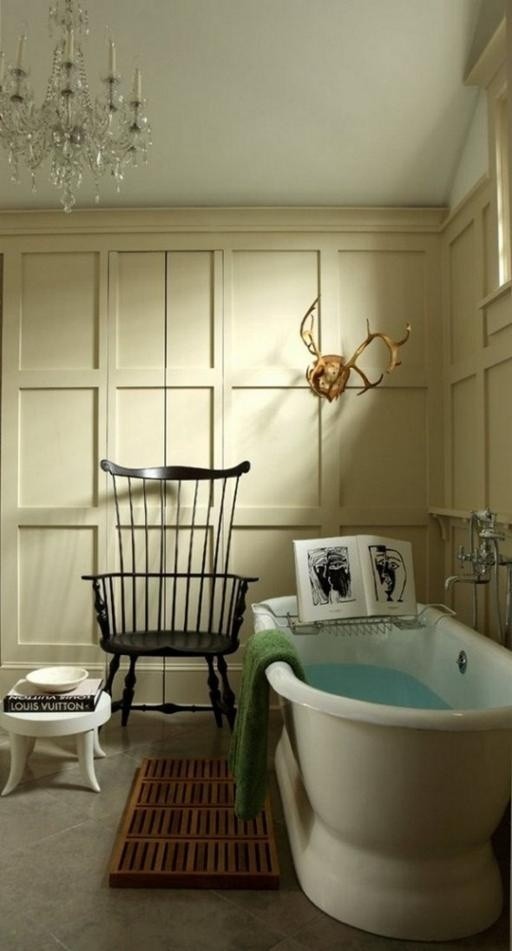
[253,591,511,941]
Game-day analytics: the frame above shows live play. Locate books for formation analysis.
[291,533,419,623]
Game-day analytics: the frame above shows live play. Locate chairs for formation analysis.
[89,459,251,732]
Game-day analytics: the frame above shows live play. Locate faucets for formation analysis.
[443,573,491,596]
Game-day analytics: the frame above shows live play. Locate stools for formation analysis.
[1,689,112,796]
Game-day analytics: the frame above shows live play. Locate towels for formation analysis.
[227,627,308,818]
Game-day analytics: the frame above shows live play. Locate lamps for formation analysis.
[2,2,153,217]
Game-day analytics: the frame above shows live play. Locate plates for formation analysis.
[26,664,89,693]
